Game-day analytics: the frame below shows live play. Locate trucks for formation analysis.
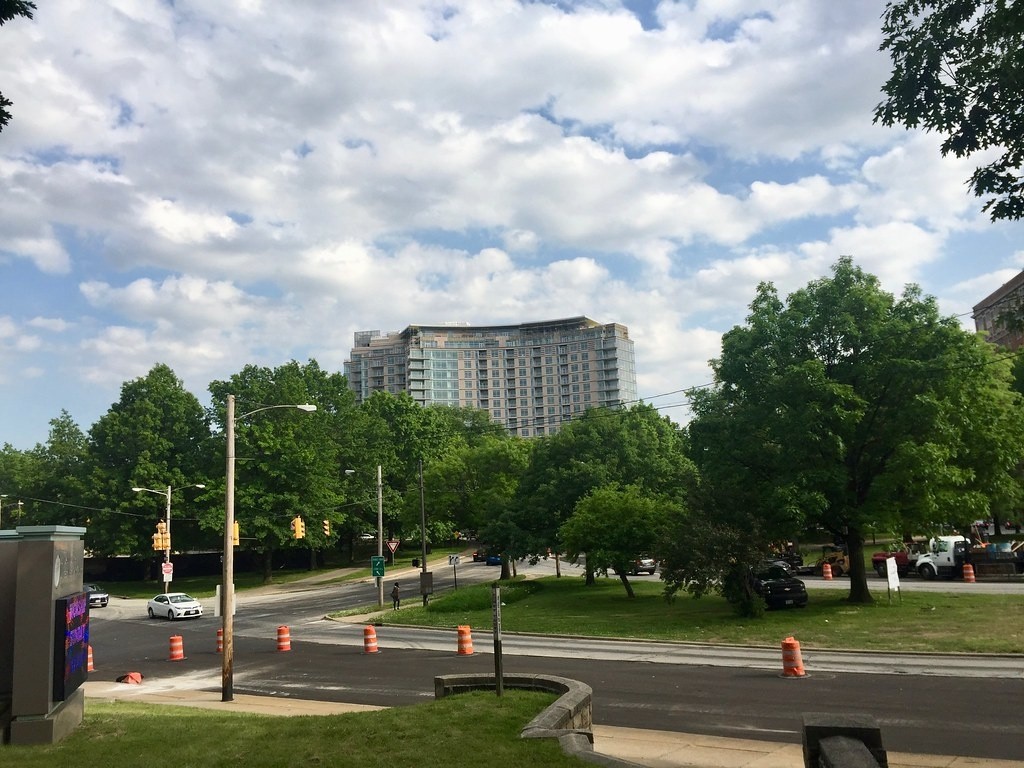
[916,520,1024,580]
[871,542,926,577]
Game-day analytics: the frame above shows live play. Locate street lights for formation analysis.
[218,394,319,703]
[344,464,385,608]
[131,484,207,594]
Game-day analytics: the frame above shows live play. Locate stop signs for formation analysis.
[161,563,174,574]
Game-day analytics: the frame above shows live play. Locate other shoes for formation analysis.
[394,609,396,610]
[397,608,399,610]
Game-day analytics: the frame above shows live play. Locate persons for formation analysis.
[392,581,400,610]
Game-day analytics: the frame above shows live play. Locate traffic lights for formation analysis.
[322,520,331,539]
[151,533,162,551]
[298,518,306,539]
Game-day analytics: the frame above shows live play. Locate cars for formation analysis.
[613,557,656,576]
[472,548,487,562]
[485,546,502,566]
[357,532,375,540]
[83,584,110,607]
[147,592,204,621]
[743,561,809,611]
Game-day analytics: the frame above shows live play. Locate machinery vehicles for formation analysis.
[792,540,850,579]
